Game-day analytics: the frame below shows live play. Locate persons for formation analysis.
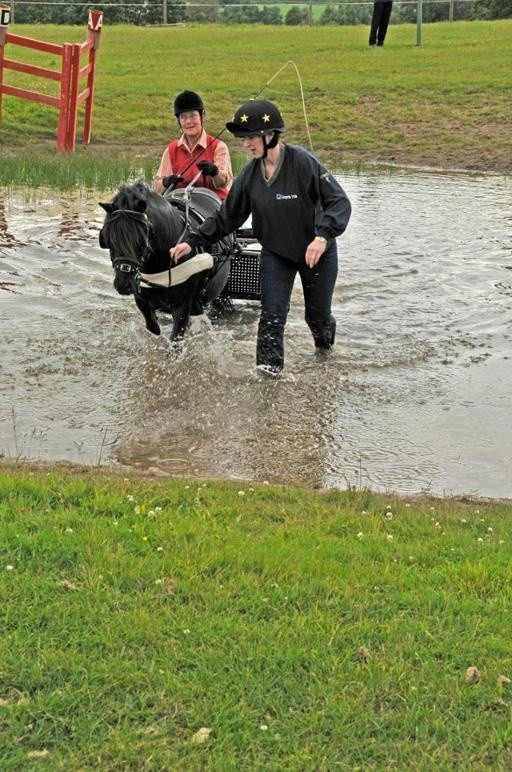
[153,93,234,224]
[168,98,352,379]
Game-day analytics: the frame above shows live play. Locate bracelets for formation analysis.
[315,236,328,245]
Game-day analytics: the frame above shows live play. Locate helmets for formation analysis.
[225,99,285,138]
[173,90,204,116]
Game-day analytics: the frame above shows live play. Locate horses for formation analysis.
[97,179,235,358]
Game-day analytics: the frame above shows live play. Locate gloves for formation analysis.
[197,160,219,179]
[163,175,185,190]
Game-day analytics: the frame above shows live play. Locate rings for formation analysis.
[312,250,316,255]
